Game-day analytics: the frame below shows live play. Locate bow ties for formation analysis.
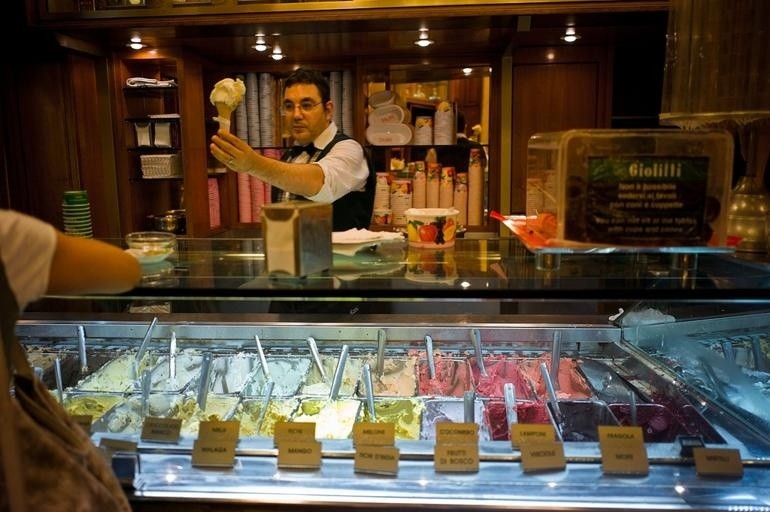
[289,143,314,158]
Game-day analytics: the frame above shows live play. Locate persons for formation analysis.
[0,209,142,385]
[209,67,376,231]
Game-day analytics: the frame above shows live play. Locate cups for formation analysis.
[236,72,354,148]
[372,147,484,229]
[403,208,460,249]
[404,246,460,284]
[413,100,456,145]
[237,149,284,223]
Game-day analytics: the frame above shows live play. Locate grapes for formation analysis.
[434,250,446,280]
[431,216,446,245]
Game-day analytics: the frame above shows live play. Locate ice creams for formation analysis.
[208,76,248,113]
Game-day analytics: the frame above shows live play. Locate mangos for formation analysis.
[444,251,456,277]
[442,219,457,242]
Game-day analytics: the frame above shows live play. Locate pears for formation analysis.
[408,220,425,241]
[407,251,424,275]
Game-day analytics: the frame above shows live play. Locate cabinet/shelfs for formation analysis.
[14,295,770,510]
[124,85,183,181]
[219,61,501,232]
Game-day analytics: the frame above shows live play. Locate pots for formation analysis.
[147,208,187,235]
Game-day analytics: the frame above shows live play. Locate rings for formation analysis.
[227,156,234,165]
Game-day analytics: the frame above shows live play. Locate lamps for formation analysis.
[414,39,435,48]
[559,35,582,42]
[126,43,147,50]
[251,45,272,52]
[268,53,287,61]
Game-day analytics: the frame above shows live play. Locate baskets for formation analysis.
[140,154,180,179]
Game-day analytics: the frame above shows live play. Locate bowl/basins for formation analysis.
[366,90,412,146]
[62,189,93,240]
[126,232,176,263]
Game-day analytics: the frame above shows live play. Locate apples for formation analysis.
[419,225,438,242]
[419,252,437,272]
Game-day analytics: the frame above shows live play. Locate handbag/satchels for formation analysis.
[0,369,131,512]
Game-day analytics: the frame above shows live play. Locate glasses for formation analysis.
[283,101,323,110]
